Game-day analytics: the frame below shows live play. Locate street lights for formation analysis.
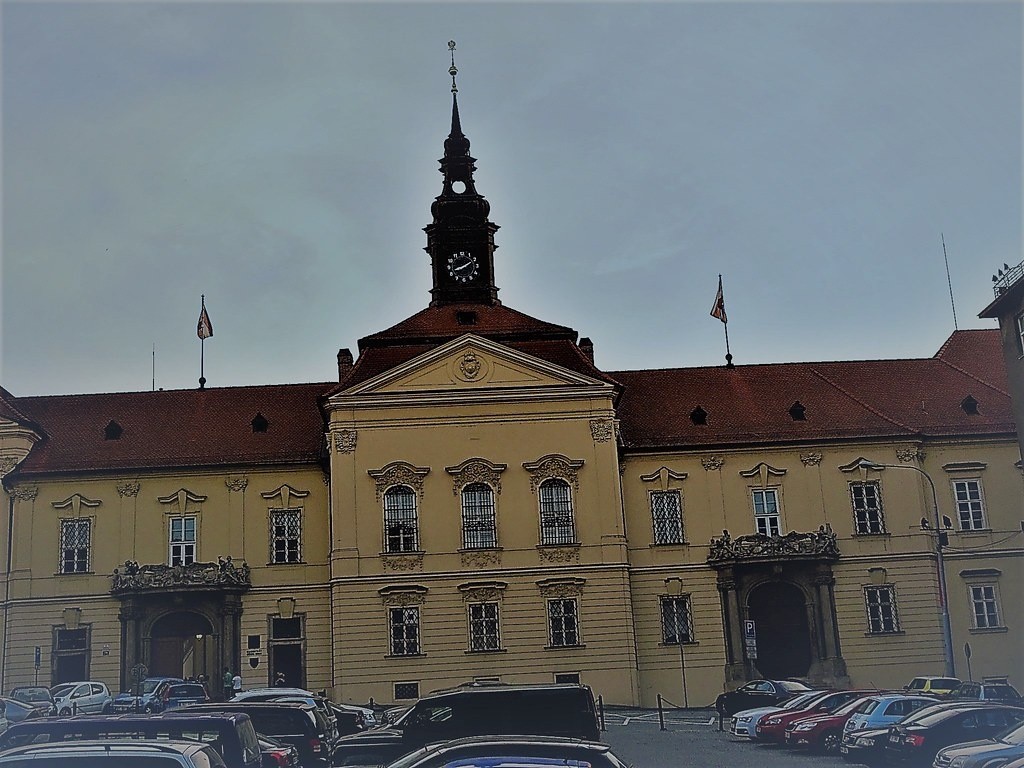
[859,459,955,675]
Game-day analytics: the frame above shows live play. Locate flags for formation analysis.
[709,277,728,322]
[197,299,213,339]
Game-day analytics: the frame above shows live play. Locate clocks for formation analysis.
[446,252,480,283]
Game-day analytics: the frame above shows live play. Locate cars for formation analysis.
[0,672,1024,768]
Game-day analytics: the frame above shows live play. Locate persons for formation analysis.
[276,672,286,687]
[219,669,244,701]
